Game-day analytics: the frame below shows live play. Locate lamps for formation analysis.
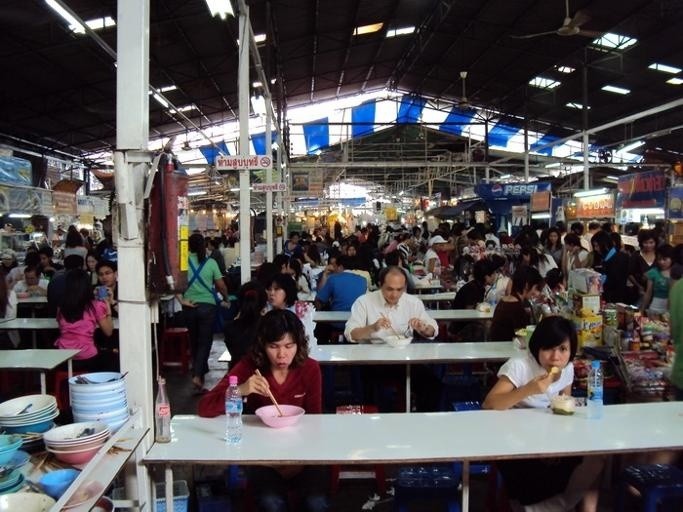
[43,0,266,118]
[351,17,418,40]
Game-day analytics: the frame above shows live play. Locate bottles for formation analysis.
[154,379,171,443]
[226,376,244,443]
[489,286,497,313]
[311,278,316,297]
[587,360,604,409]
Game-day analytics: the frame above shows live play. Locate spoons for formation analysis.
[548,366,560,375]
[404,322,412,337]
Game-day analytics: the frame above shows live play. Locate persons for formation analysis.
[1,223,682,411]
[627,244,683,499]
[196,309,334,512]
[480,316,606,512]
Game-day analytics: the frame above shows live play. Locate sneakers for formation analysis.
[192,376,202,389]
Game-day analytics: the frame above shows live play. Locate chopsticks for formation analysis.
[255,369,282,415]
[381,312,399,338]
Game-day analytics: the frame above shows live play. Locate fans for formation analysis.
[449,71,483,109]
[509,1,601,41]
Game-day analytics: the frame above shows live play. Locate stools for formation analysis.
[55,369,85,409]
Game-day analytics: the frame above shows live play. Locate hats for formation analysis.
[430,236,449,244]
[1,248,15,260]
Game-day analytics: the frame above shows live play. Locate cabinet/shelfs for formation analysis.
[20,404,151,512]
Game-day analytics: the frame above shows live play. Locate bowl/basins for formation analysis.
[255,404,306,429]
[1,372,129,512]
[551,395,576,415]
[384,336,414,349]
[17,292,29,297]
